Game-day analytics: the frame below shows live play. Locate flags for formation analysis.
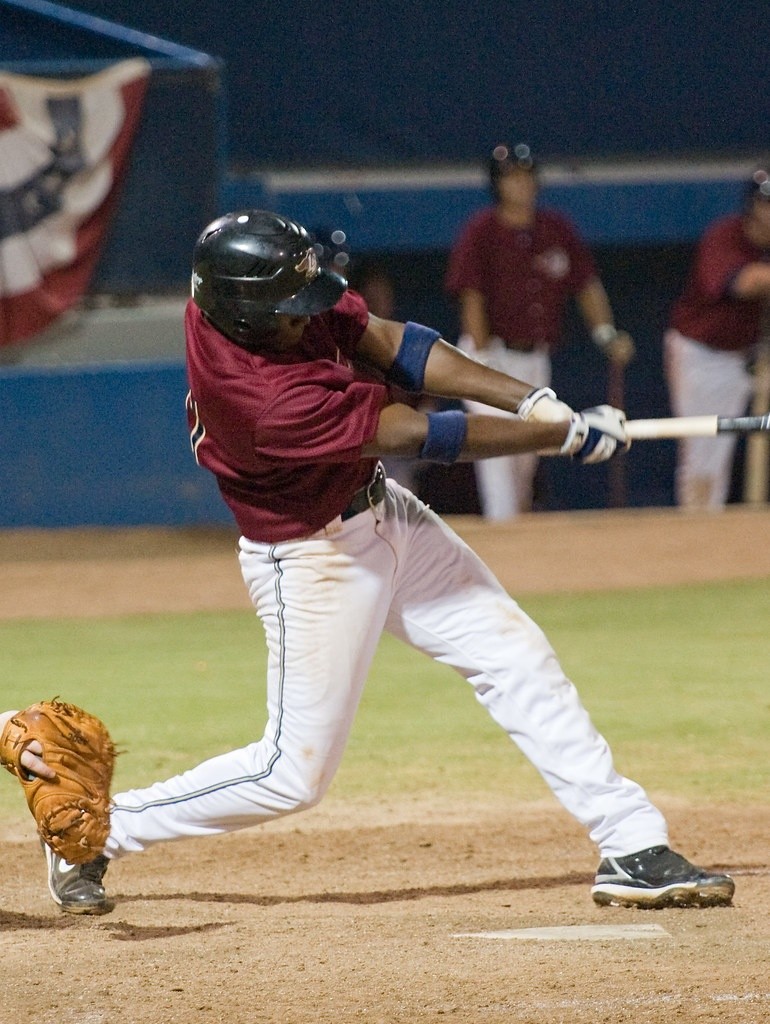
[0,57,152,350]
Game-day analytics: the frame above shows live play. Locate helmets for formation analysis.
[189,208,350,350]
[489,138,538,179]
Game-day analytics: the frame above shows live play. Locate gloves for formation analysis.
[576,403,633,457]
[559,414,618,465]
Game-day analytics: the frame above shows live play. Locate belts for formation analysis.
[339,458,387,524]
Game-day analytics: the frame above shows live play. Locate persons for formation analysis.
[0,696,116,865]
[304,137,770,519]
[44,212,738,910]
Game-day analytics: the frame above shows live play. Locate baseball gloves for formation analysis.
[0,700,120,859]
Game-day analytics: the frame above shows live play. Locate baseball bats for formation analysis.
[575,415,768,443]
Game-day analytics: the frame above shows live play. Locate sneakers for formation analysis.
[48,832,110,915]
[591,847,736,908]
